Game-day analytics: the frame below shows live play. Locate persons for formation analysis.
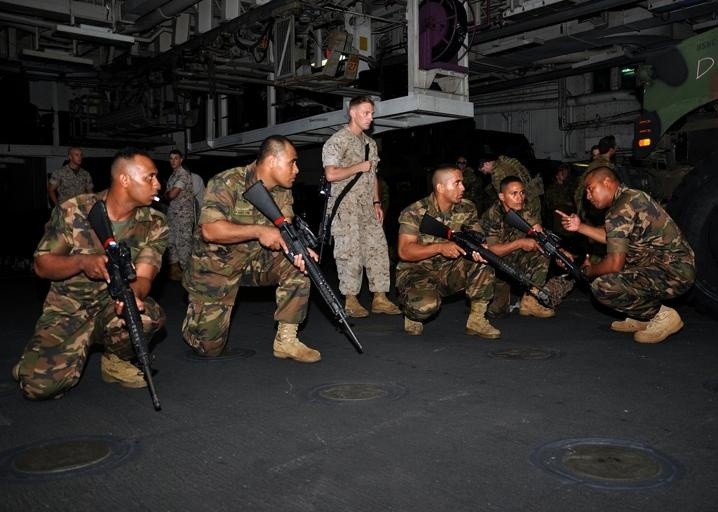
[376,177,392,217]
[554,166,697,344]
[573,134,623,252]
[542,162,577,217]
[455,157,486,218]
[590,145,600,161]
[184,166,205,212]
[475,155,544,227]
[479,175,556,318]
[47,146,94,205]
[182,134,322,366]
[392,166,502,339]
[164,150,197,282]
[321,94,403,316]
[10,146,174,404]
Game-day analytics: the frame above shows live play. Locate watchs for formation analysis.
[373,201,382,206]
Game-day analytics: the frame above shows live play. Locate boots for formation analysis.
[466,310,502,338]
[405,316,424,334]
[520,291,555,317]
[611,316,650,332]
[635,304,684,343]
[273,323,321,361]
[371,291,402,314]
[345,294,369,317]
[102,354,147,388]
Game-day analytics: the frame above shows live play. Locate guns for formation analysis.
[86,200,162,412]
[499,207,590,285]
[419,212,554,309]
[240,178,367,355]
[318,174,329,266]
